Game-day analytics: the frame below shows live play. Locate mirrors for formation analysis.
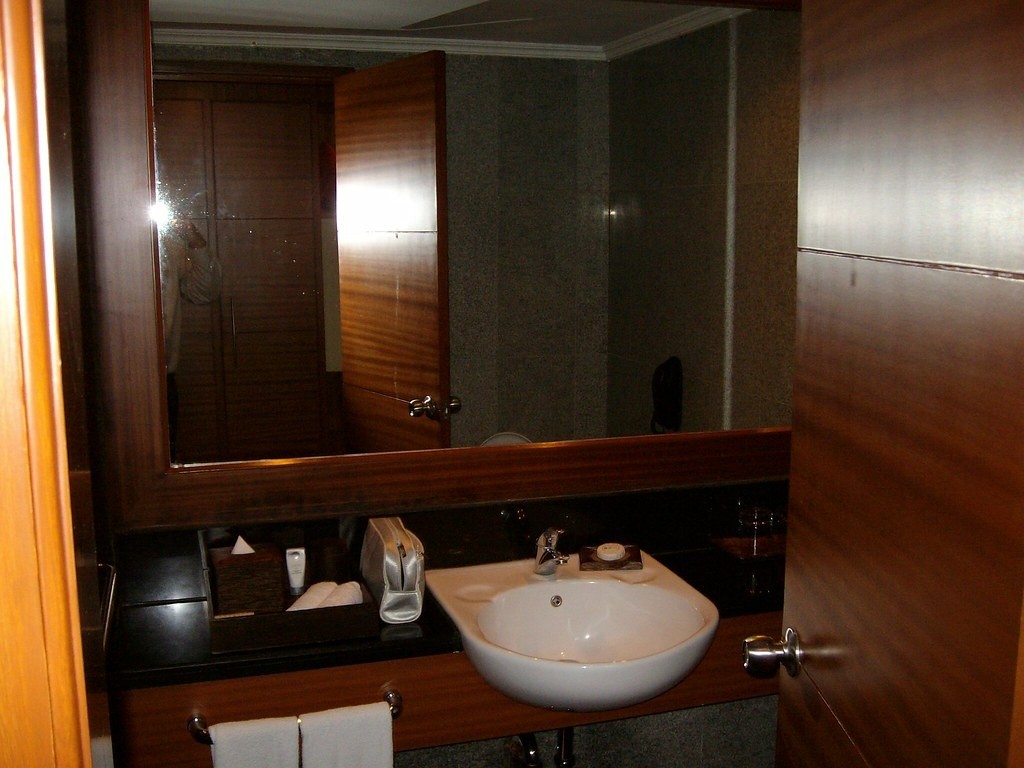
[147,0,799,470]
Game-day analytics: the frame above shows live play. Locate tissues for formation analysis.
[208,532,289,618]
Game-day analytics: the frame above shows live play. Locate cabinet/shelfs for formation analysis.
[152,77,328,463]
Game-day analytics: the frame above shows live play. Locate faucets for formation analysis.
[532,527,572,577]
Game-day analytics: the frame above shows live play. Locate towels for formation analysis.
[300,700,395,768]
[208,714,300,768]
[285,580,339,612]
[322,581,364,608]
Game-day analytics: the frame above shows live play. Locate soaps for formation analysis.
[596,543,628,562]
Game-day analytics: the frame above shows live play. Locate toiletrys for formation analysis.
[286,547,307,597]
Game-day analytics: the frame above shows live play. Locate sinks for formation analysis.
[420,544,707,713]
